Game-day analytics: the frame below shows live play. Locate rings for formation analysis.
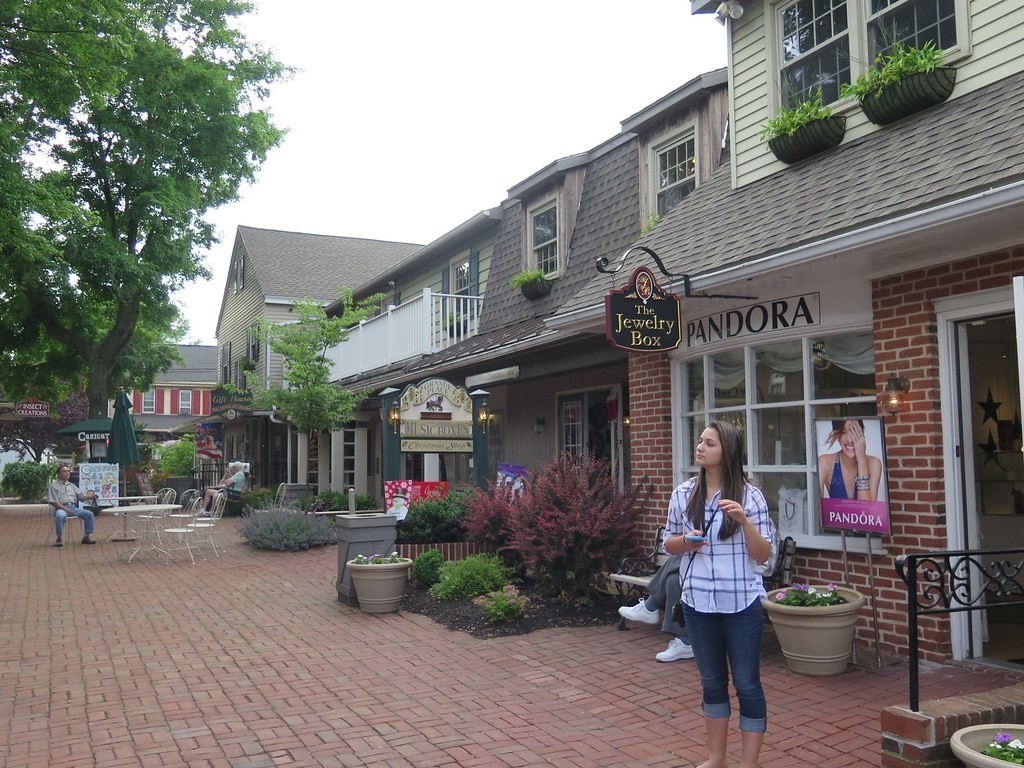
[860,433,864,437]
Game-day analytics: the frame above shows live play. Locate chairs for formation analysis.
[46,481,287,565]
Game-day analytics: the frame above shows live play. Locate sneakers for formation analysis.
[617,597,660,624]
[655,638,694,662]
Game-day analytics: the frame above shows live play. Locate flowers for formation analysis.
[356,552,402,565]
[981,730,1024,765]
[777,583,844,608]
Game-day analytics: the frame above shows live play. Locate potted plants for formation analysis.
[509,269,552,298]
[760,14,957,164]
[442,312,468,336]
[240,356,256,372]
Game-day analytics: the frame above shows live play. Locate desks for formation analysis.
[98,496,159,543]
[102,504,182,562]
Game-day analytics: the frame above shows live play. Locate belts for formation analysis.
[63,502,76,506]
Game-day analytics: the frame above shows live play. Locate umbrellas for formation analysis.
[53,411,144,464]
[105,385,140,505]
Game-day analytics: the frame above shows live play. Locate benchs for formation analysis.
[223,473,251,515]
[609,525,797,631]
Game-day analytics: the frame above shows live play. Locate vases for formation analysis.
[347,557,414,614]
[762,585,1024,767]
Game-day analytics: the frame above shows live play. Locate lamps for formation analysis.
[714,0,743,25]
[388,279,398,287]
[533,417,545,435]
[882,374,909,415]
[478,399,489,436]
[387,400,399,433]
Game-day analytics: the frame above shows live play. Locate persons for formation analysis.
[819,419,882,528]
[194,460,245,517]
[47,465,97,546]
[661,420,774,768]
[618,478,781,662]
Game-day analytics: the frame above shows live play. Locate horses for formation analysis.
[426,396,444,411]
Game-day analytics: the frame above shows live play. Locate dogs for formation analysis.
[201,436,217,450]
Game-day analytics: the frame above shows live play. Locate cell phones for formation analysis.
[685,535,708,542]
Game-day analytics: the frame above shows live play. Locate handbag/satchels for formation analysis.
[671,600,685,628]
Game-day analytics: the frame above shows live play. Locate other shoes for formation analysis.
[55,538,63,546]
[194,511,206,517]
[204,512,210,517]
[82,536,96,544]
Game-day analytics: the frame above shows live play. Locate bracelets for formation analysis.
[855,476,870,491]
